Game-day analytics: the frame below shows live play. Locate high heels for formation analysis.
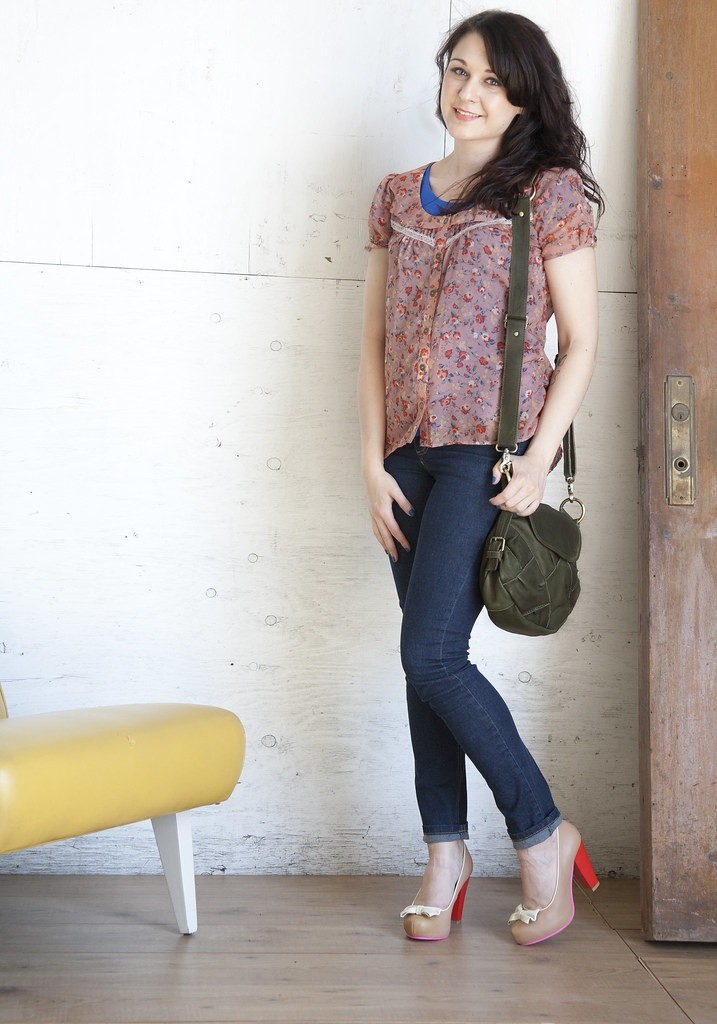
[508,820,601,946]
[400,843,473,940]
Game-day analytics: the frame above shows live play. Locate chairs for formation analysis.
[0,687,246,935]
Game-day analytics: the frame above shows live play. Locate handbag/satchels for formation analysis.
[480,470,585,636]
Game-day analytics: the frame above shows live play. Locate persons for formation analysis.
[363,13,608,947]
